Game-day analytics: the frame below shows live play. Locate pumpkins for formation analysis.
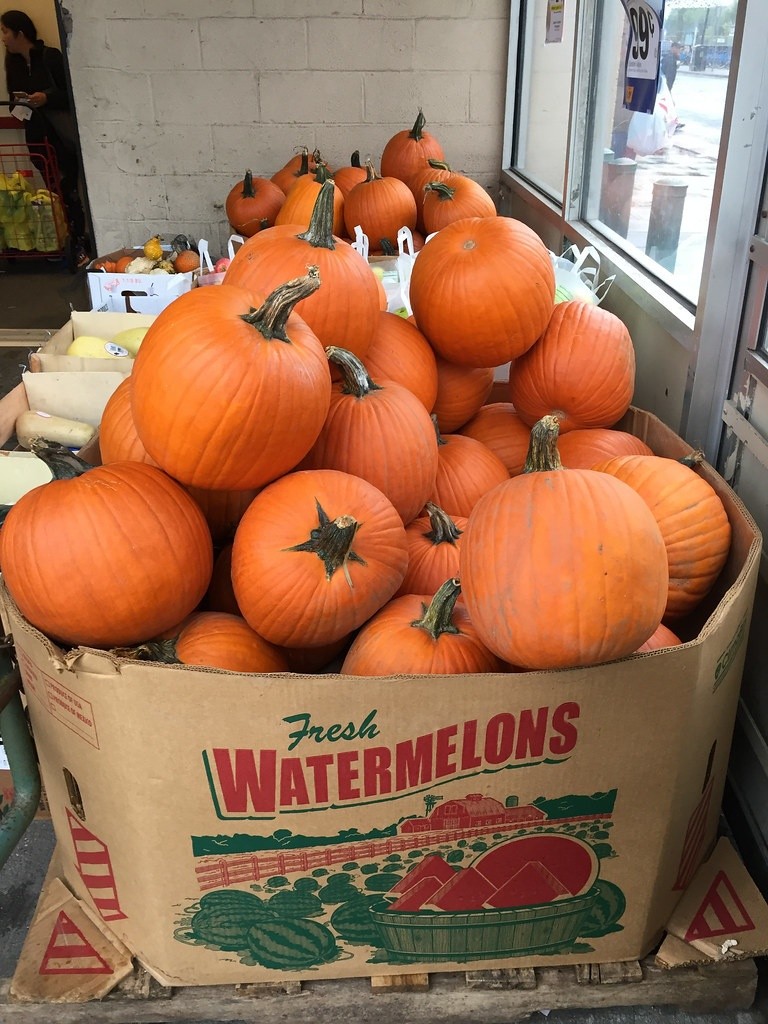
[0,113,732,673]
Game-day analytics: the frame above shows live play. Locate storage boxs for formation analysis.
[1,245,763,989]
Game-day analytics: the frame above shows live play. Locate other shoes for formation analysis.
[71,248,90,267]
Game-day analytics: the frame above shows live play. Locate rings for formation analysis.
[34,102,37,105]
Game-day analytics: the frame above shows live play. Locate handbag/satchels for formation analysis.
[351,226,618,321]
[626,79,677,154]
[197,234,245,286]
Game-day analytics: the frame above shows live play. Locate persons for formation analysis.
[0,10,94,266]
[661,42,681,92]
